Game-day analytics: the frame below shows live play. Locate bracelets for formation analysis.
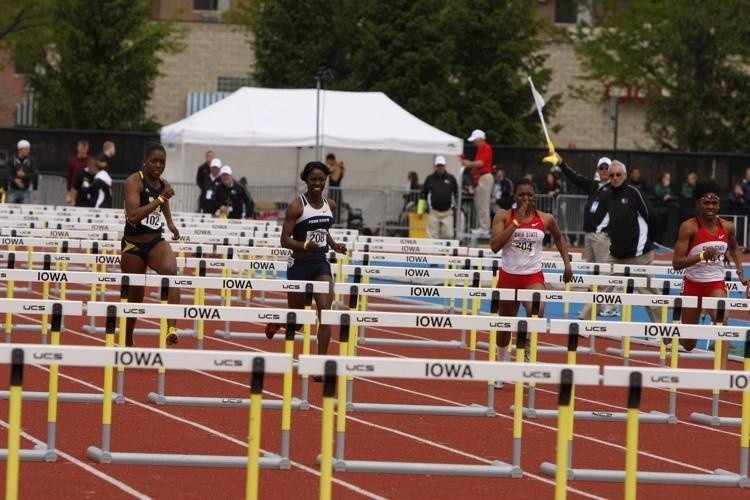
[303,240,309,252]
[158,195,169,205]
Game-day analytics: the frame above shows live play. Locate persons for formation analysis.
[264,163,347,383]
[120,144,180,345]
[325,153,345,222]
[195,151,255,219]
[1,141,39,204]
[66,138,116,207]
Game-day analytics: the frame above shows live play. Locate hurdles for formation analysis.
[0,343,294,500]
[602,364,750,500]
[87,299,318,472]
[317,309,548,480]
[1,297,83,463]
[297,352,600,500]
[1,202,750,429]
[539,318,750,488]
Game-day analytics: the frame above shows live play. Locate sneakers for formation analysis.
[166,327,178,344]
[265,323,278,338]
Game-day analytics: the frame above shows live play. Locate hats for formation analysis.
[597,157,611,167]
[209,158,232,176]
[435,157,446,166]
[467,129,485,142]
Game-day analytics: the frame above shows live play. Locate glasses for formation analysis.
[599,167,608,170]
[609,173,621,177]
